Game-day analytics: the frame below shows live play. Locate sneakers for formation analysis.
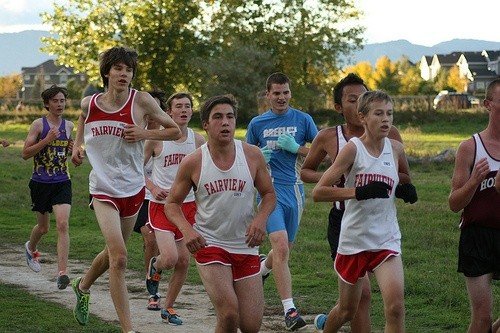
[313,314,327,333]
[258,254,270,286]
[160,307,183,326]
[285,308,306,331]
[72,277,90,326]
[146,257,162,295]
[56,271,70,290]
[148,293,161,311]
[25,240,41,273]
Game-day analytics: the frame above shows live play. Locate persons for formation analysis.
[22,86,75,290]
[496,168,500,192]
[246,73,318,331]
[447,79,500,333]
[133,90,204,326]
[164,95,277,333]
[311,90,419,333]
[71,46,183,333]
[300,74,402,333]
[0,139,9,147]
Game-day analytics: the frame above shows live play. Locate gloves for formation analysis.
[394,183,418,204]
[260,145,273,163]
[355,181,392,200]
[275,132,300,154]
